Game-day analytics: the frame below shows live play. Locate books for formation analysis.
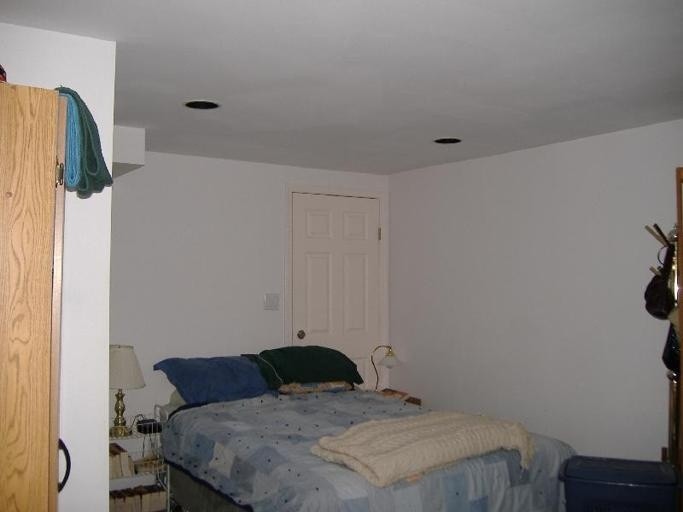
[381,386,411,400]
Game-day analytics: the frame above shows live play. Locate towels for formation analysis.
[53,86,113,199]
[57,93,82,189]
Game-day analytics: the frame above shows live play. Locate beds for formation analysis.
[154,387,576,512]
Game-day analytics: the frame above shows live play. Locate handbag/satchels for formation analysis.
[645,245,676,319]
[663,322,680,375]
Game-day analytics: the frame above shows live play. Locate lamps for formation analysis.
[108,343,146,436]
[369,343,403,392]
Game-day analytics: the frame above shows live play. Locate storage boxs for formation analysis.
[558,454,677,511]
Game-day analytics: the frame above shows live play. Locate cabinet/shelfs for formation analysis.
[0,81,71,511]
[109,403,172,511]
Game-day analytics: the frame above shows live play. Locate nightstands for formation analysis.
[372,387,423,406]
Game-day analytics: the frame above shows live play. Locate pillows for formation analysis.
[152,345,365,407]
